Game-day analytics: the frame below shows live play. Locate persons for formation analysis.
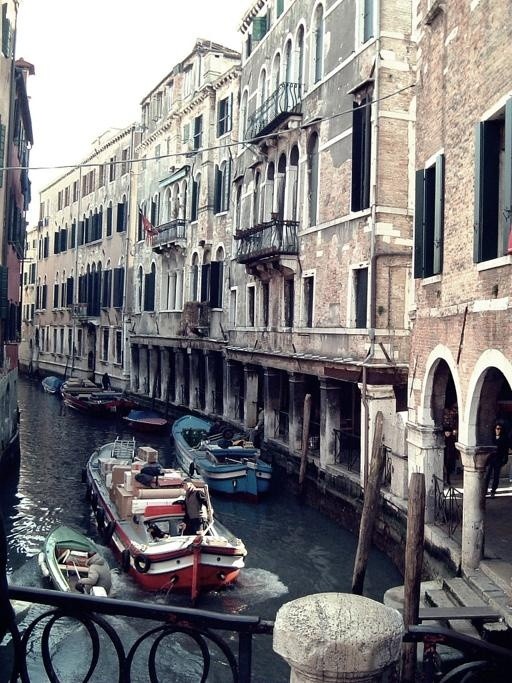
[484,423,509,498]
[77,551,111,595]
[444,428,458,488]
[103,373,112,390]
[208,419,221,434]
[255,404,265,432]
[181,476,207,536]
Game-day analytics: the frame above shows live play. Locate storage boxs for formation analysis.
[96,458,134,520]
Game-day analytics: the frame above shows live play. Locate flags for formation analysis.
[141,216,157,238]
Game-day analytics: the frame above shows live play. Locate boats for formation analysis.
[38,525,111,598]
[41,375,272,496]
[86,442,247,600]
